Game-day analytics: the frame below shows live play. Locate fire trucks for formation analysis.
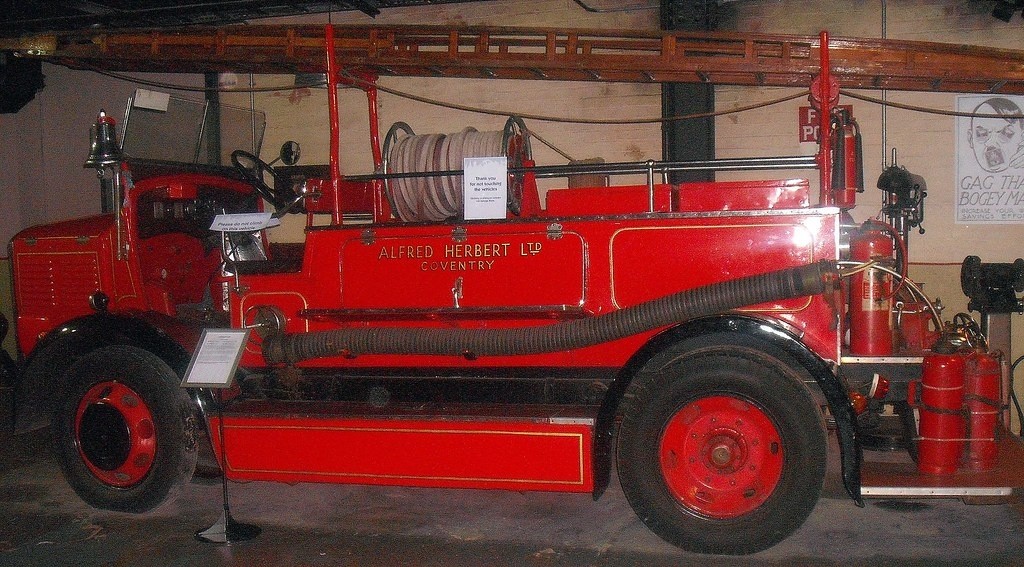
[0,0,1024,558]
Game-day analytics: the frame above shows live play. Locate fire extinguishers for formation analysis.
[916,335,966,477]
[967,347,1006,470]
[847,215,908,356]
[831,107,864,207]
